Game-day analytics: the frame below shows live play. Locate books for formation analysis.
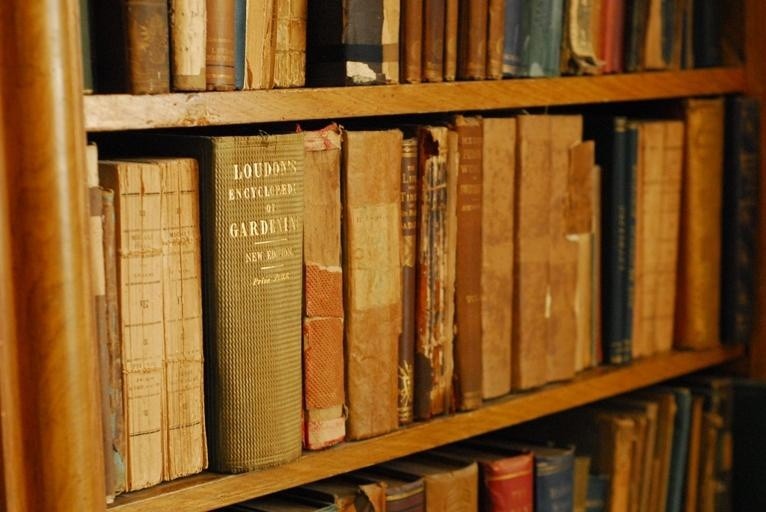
[253,375,764,511]
[85,92,761,491]
[89,1,749,95]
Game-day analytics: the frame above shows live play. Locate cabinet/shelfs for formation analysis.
[0,1,766,512]
